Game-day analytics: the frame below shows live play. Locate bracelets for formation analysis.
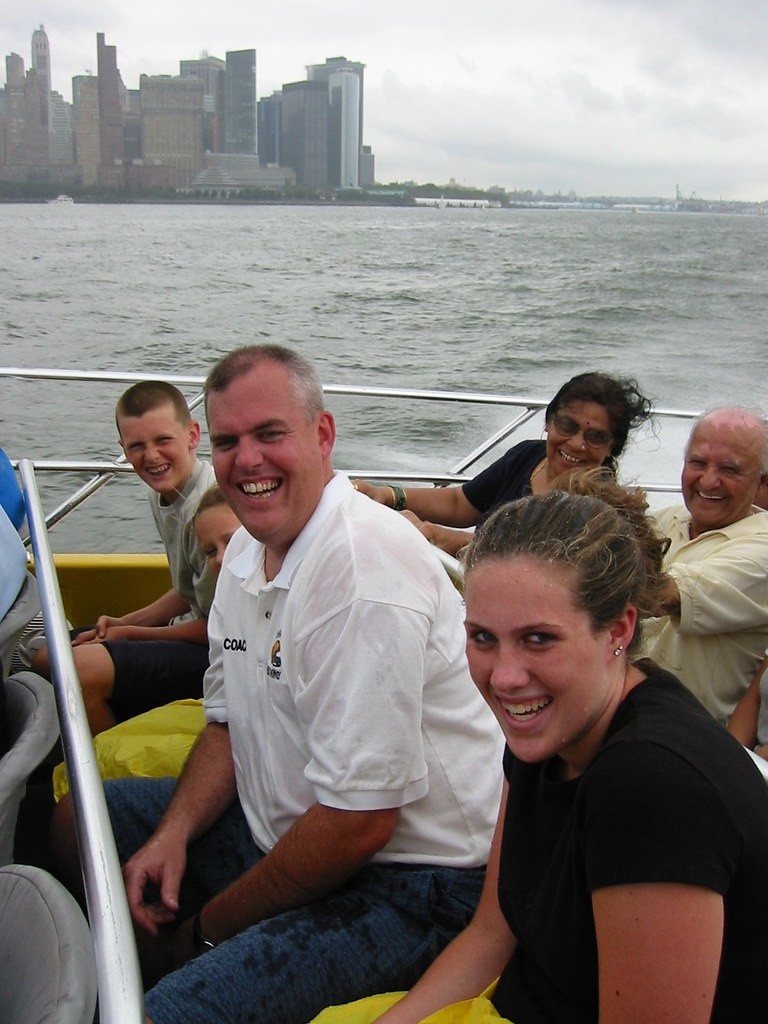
[386,485,407,512]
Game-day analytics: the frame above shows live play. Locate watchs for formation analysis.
[190,911,215,951]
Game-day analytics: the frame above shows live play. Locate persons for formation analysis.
[44,342,509,1022]
[458,405,767,724]
[724,649,768,760]
[350,368,650,554]
[32,380,223,736]
[370,467,766,1021]
[189,482,243,579]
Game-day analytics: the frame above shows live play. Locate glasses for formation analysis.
[548,408,614,446]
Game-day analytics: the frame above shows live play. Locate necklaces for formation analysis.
[530,457,547,481]
